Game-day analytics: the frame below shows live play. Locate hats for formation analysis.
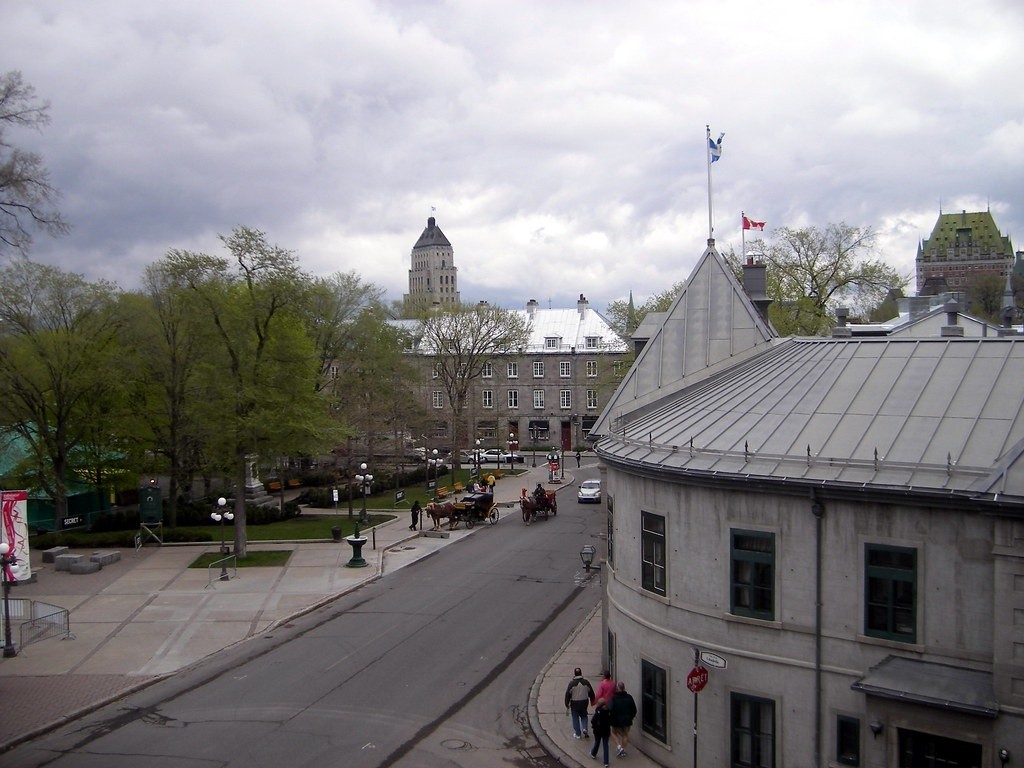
[537,484,541,486]
[468,480,472,483]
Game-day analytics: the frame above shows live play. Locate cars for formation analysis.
[443,450,484,464]
[577,478,600,503]
[479,449,518,462]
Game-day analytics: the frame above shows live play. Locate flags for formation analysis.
[742,217,767,231]
[708,131,725,163]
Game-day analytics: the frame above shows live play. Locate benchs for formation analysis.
[336,472,344,481]
[55,554,84,571]
[493,470,505,480]
[454,482,466,494]
[89,550,122,566]
[437,486,454,500]
[482,472,490,482]
[288,479,300,489]
[346,470,357,478]
[269,482,286,492]
[42,547,68,563]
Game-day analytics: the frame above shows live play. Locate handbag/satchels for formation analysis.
[493,482,496,486]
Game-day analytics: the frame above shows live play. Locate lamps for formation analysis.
[585,413,588,416]
[551,412,553,415]
[998,745,1009,768]
[869,717,882,741]
[580,544,601,573]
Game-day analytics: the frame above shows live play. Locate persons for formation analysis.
[589,671,637,767]
[409,501,421,531]
[467,473,495,493]
[576,452,580,467]
[534,484,546,496]
[565,668,595,739]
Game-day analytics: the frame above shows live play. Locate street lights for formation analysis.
[506,433,520,471]
[428,449,443,507]
[355,464,373,523]
[1,542,20,656]
[209,497,236,584]
[471,440,486,483]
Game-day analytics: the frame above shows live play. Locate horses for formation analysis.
[518,496,537,526]
[425,501,455,530]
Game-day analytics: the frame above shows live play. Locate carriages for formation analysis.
[425,488,499,529]
[519,488,558,526]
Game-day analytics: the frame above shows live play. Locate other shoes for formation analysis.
[414,526,416,531]
[582,728,590,739]
[589,752,596,759]
[409,526,413,531]
[605,763,610,767]
[573,733,581,739]
[615,746,625,758]
[618,751,628,757]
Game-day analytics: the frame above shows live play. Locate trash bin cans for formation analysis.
[332,526,342,541]
[518,457,524,463]
